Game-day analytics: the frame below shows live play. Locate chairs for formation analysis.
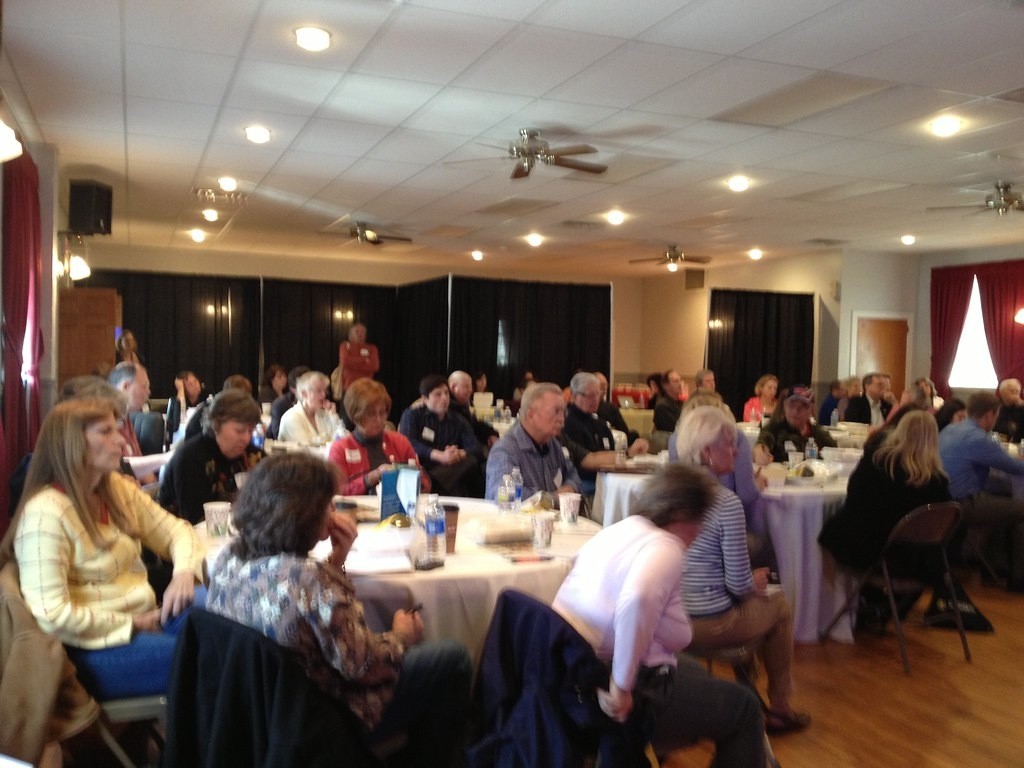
[682,642,784,768]
[120,451,173,488]
[164,608,409,768]
[0,561,172,768]
[470,590,660,768]
[819,500,973,677]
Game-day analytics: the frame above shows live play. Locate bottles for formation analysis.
[830,407,839,427]
[425,493,446,564]
[251,423,265,451]
[494,398,512,424]
[510,466,523,509]
[751,406,767,427]
[615,430,628,465]
[805,437,819,462]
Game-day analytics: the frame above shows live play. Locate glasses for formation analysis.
[576,389,600,400]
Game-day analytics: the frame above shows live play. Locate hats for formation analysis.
[785,384,815,404]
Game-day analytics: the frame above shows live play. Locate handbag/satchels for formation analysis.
[925,575,994,632]
[331,341,350,399]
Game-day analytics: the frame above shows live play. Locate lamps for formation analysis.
[62,244,91,283]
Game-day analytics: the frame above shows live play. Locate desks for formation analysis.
[116,403,1024,668]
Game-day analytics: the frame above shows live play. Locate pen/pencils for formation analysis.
[761,443,765,452]
[406,603,423,615]
[511,556,554,562]
[755,467,762,479]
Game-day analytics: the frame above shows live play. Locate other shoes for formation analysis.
[1007,575,1024,589]
[866,605,888,634]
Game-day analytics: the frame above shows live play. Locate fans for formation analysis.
[628,243,710,266]
[314,220,413,250]
[442,126,609,179]
[925,181,1024,219]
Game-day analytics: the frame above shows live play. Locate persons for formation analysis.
[340,322,380,432]
[204,452,473,768]
[117,330,140,363]
[819,402,967,632]
[330,380,431,495]
[743,374,779,421]
[486,383,589,511]
[753,401,838,466]
[938,391,1024,587]
[551,461,767,768]
[690,368,742,422]
[398,366,500,499]
[260,365,351,445]
[559,372,649,496]
[993,379,1024,443]
[166,371,212,450]
[777,373,897,425]
[668,389,779,584]
[185,375,252,441]
[653,369,684,432]
[159,389,269,573]
[107,363,159,484]
[676,406,811,737]
[888,376,966,436]
[647,372,663,409]
[0,375,201,702]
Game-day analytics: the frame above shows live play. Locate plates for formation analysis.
[788,471,831,486]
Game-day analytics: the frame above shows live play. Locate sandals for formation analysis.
[767,710,810,734]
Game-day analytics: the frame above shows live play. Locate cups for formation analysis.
[530,512,554,549]
[498,474,516,513]
[203,501,231,538]
[439,501,459,553]
[558,492,581,524]
[788,452,804,469]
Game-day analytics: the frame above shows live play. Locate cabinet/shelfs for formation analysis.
[58,286,120,399]
